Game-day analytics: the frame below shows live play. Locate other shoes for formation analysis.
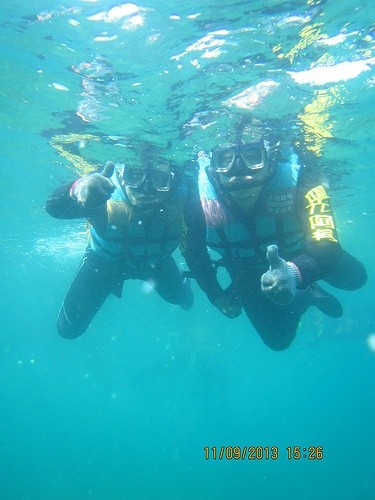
[309,283,343,318]
[177,267,192,311]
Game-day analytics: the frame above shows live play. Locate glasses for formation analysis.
[122,165,174,192]
[212,139,266,173]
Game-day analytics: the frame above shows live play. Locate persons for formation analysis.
[199,138,368,351]
[46,161,241,339]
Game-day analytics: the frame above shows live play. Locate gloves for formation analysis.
[79,160,115,208]
[260,245,302,306]
